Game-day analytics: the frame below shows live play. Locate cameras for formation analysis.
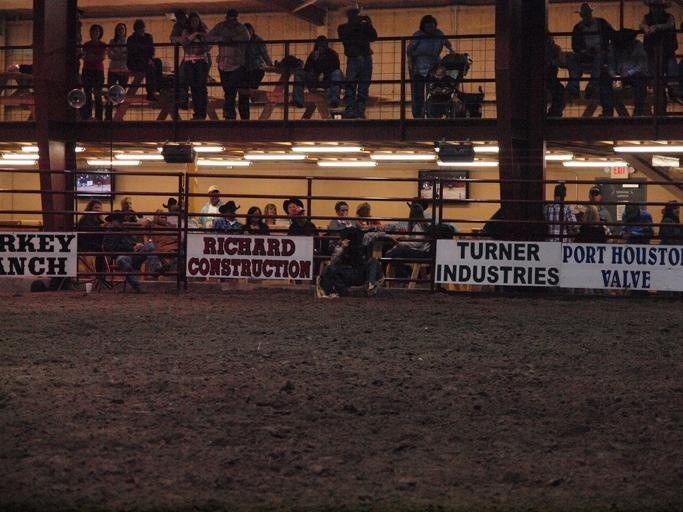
[360,16,368,20]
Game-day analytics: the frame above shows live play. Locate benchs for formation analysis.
[0,64,348,121]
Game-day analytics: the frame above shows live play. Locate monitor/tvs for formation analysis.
[418,169,470,205]
[74,168,115,201]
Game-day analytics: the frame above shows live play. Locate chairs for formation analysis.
[70,222,432,293]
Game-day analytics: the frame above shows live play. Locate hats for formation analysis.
[574,4,592,15]
[339,2,364,17]
[219,201,241,212]
[283,199,305,215]
[207,185,220,194]
[645,0,672,8]
[163,198,177,208]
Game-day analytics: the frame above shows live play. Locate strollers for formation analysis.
[427,51,485,119]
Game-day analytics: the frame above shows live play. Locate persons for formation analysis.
[283,197,455,295]
[407,15,455,120]
[428,66,456,95]
[104,23,130,120]
[127,18,167,101]
[483,185,615,294]
[304,35,343,108]
[81,24,106,120]
[620,203,654,294]
[75,197,199,295]
[657,200,682,295]
[544,1,679,118]
[337,8,377,120]
[193,186,277,236]
[169,8,273,120]
